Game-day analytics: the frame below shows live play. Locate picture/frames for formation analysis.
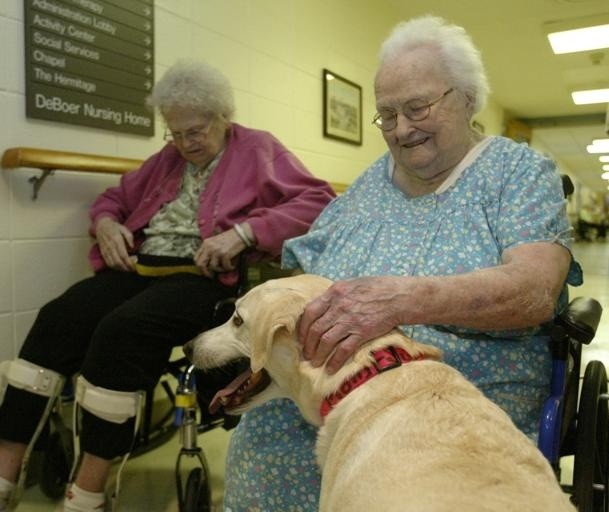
[321,68,363,147]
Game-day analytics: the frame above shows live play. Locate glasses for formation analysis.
[371,85,461,132]
[163,114,215,143]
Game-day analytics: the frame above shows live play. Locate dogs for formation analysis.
[180,274,582,512]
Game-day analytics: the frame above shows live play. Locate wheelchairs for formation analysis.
[11,249,258,511]
[526,176,607,510]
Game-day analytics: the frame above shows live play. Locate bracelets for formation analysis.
[233,220,259,253]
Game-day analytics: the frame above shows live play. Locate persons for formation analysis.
[222,11,586,511]
[0,53,339,510]
